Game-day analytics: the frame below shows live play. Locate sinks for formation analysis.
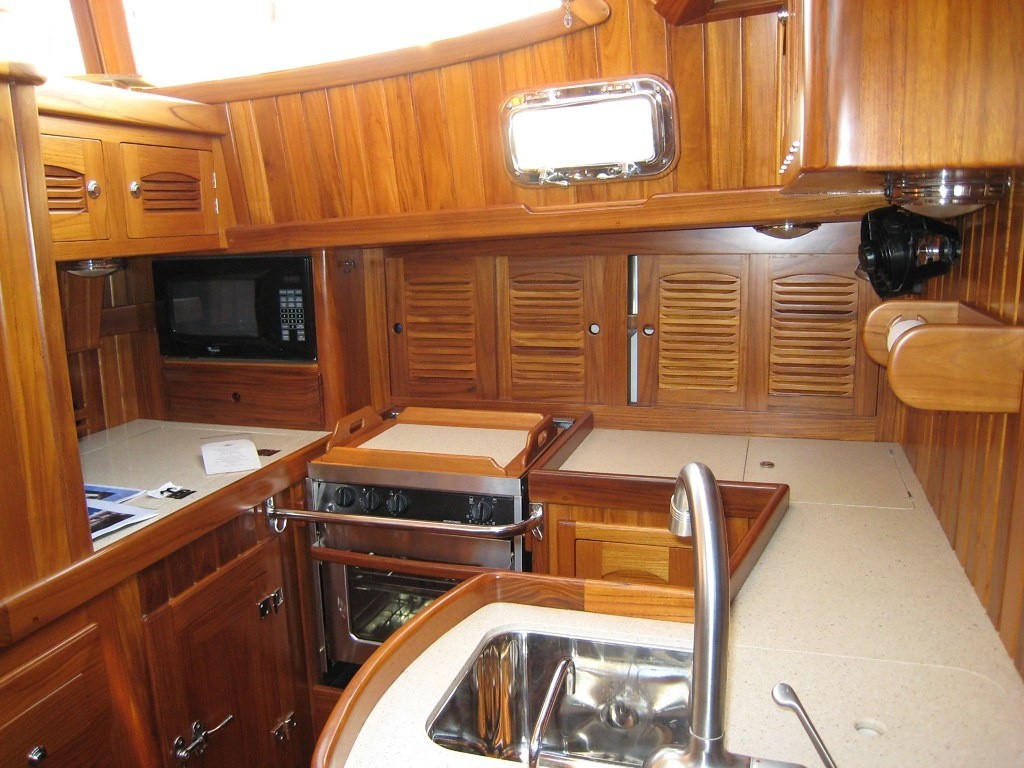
[428,630,692,768]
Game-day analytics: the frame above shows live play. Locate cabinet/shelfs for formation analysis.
[0,487,318,768]
[1,1,1024,663]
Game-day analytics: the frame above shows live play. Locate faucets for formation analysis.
[669,461,726,767]
[529,659,576,767]
